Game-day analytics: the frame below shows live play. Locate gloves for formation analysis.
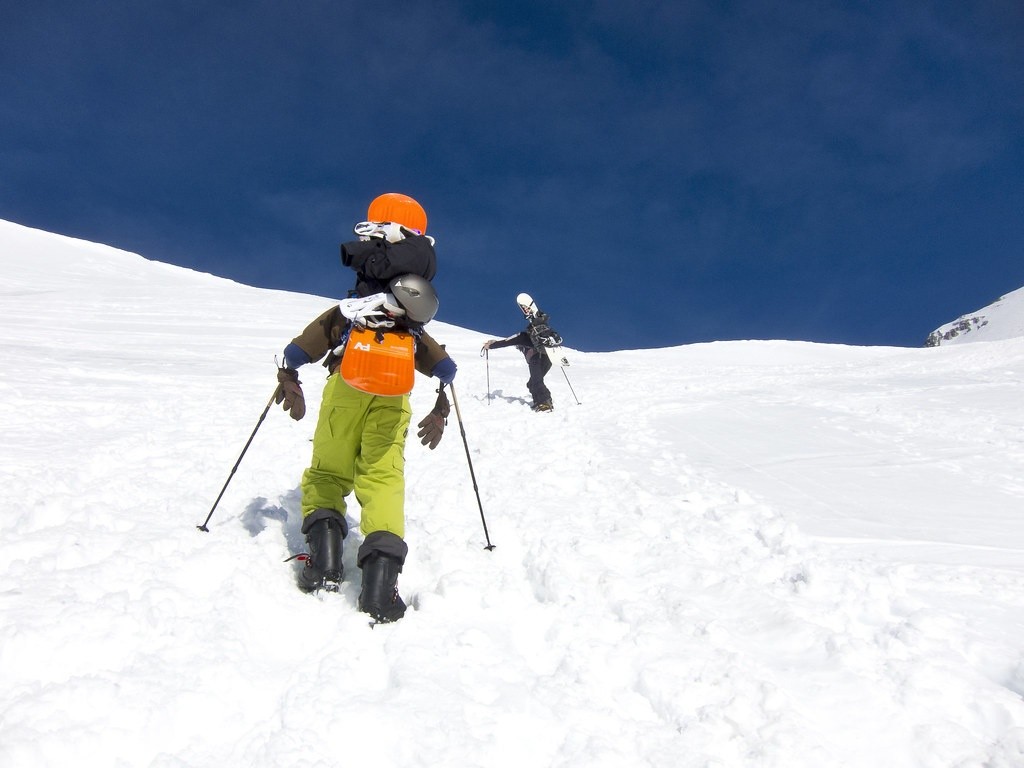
[275,368,306,420]
[485,340,492,350]
[418,392,450,449]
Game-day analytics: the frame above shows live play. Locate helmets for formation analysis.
[390,273,440,322]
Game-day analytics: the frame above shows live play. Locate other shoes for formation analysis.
[531,399,554,412]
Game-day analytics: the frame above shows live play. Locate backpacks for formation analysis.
[341,235,437,342]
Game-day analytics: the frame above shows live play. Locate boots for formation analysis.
[357,552,406,625]
[284,518,347,595]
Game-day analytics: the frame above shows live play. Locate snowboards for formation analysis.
[338,191,429,398]
[516,292,571,367]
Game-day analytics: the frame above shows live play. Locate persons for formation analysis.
[273,294,459,626]
[479,332,555,411]
